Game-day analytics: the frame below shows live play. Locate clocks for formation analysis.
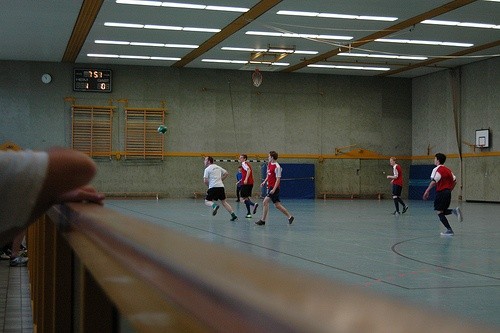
[41,73,53,84]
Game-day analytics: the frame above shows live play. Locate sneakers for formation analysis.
[441,231,454,236]
[453,208,463,222]
[288,217,294,224]
[253,203,258,214]
[392,211,400,215]
[402,206,409,214]
[230,216,237,221]
[255,219,265,225]
[213,205,219,215]
[246,214,251,218]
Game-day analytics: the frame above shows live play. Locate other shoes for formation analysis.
[0,253,10,259]
[236,199,240,202]
[9,257,29,266]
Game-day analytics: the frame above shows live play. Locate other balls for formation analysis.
[157,124,168,135]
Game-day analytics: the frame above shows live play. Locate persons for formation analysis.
[203,157,237,221]
[387,157,408,215]
[236,155,258,217]
[423,153,463,236]
[255,151,295,225]
[0,150,105,267]
[236,165,242,202]
